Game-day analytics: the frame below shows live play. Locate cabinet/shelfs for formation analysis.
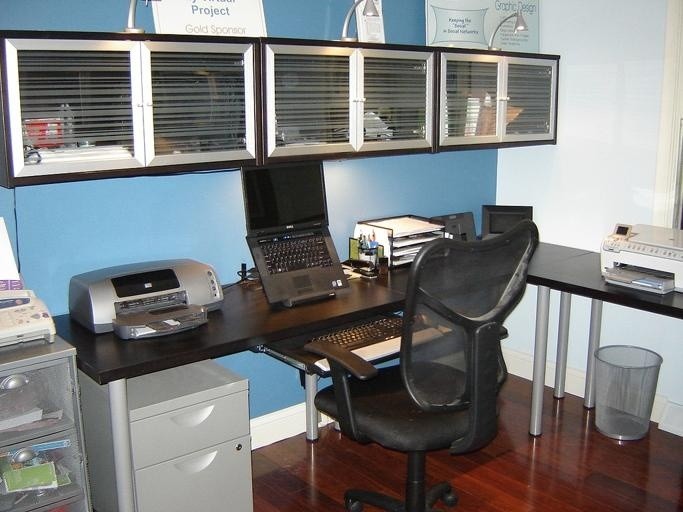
[438,45,563,154]
[126,355,256,512]
[0,29,260,190]
[0,332,94,511]
[263,35,436,164]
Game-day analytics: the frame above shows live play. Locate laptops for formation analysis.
[241,158,350,307]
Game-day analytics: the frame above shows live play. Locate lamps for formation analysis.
[337,0,380,41]
[486,10,532,52]
[121,0,162,35]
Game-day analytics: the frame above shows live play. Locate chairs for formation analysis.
[300,217,540,512]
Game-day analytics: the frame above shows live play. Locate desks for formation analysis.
[52,238,592,512]
[527,251,683,437]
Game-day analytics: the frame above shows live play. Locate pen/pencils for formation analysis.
[358,229,376,250]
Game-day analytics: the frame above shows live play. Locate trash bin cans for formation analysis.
[595,345,663,440]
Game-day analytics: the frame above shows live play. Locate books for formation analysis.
[365,219,464,265]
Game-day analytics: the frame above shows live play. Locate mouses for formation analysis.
[418,310,433,328]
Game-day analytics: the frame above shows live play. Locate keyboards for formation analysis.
[309,315,420,350]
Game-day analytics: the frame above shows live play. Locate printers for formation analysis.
[600,223,682,294]
[70,259,224,340]
